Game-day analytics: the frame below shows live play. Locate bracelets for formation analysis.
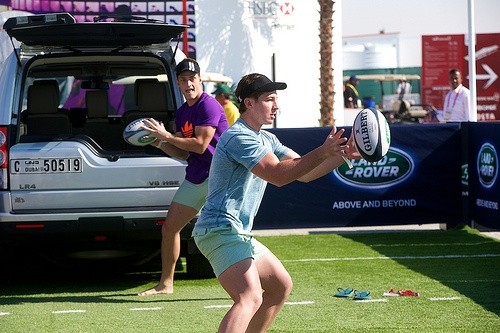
[341,150,353,169]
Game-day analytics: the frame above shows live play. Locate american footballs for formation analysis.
[123,118,159,146]
[352,105,392,162]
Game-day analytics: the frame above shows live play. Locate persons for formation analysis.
[343,75,362,108]
[427,70,469,122]
[211,83,241,127]
[139,59,229,297]
[398,80,410,94]
[191,73,361,333]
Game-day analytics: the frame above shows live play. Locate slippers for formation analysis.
[333,287,353,297]
[353,290,370,300]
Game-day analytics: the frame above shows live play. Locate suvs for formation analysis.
[0,13,213,280]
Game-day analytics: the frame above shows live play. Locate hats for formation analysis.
[210,83,231,97]
[237,76,287,103]
[176,62,201,75]
[349,75,360,82]
